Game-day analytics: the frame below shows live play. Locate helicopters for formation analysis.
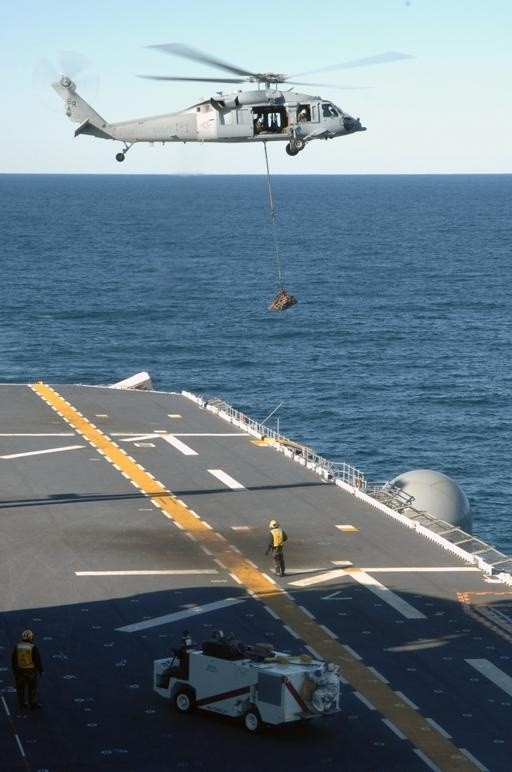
[48,39,416,158]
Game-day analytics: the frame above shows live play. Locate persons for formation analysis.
[297,108,310,122]
[11,629,46,715]
[264,519,288,577]
[158,633,201,689]
[255,117,265,134]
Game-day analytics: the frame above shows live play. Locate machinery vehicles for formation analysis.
[150,640,343,734]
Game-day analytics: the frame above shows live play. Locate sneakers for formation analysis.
[275,573,285,576]
[31,703,42,708]
[19,703,26,710]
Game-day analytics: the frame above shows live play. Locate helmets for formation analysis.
[22,630,33,639]
[269,520,279,528]
